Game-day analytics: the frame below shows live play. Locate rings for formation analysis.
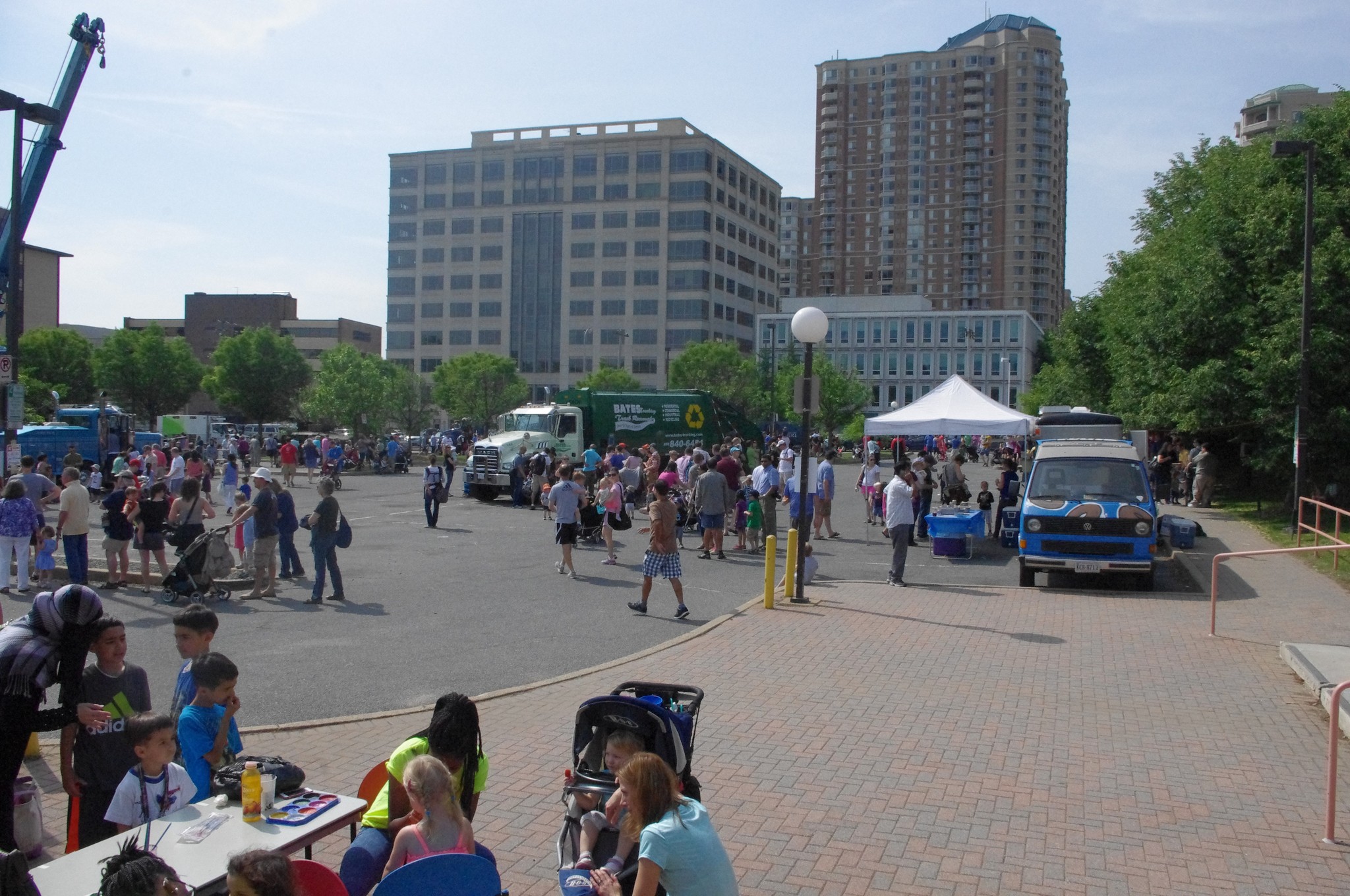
[92,720,97,726]
[597,880,602,884]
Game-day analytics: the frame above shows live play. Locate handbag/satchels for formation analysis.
[216,482,225,496]
[212,753,306,801]
[335,503,353,551]
[162,524,188,547]
[606,504,634,531]
[942,482,973,503]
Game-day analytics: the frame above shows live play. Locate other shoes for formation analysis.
[36,581,54,590]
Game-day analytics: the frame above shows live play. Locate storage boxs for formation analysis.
[1003,506,1021,528]
[933,532,966,556]
[1169,518,1197,548]
[1000,528,1019,549]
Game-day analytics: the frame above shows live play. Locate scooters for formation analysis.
[990,448,1005,466]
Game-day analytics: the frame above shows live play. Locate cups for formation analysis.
[138,844,158,857]
[980,495,986,502]
[953,507,959,517]
[932,507,938,517]
[937,509,941,517]
[260,774,277,814]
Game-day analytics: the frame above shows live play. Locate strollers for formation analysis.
[556,677,703,896]
[965,445,979,462]
[936,446,948,461]
[573,494,604,547]
[669,487,700,532]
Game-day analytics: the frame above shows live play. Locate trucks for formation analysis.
[0,391,166,491]
[292,432,331,443]
[156,415,251,451]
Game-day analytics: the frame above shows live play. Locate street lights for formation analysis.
[792,305,829,603]
[1000,358,1010,408]
[583,329,591,376]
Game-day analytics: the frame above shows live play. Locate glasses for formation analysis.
[164,877,196,896]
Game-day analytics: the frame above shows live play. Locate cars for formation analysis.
[905,435,952,453]
[333,423,461,449]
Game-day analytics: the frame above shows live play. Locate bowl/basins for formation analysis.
[957,512,964,518]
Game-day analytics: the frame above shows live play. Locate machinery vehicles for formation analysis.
[462,387,766,506]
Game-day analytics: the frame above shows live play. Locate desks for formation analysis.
[924,509,985,560]
[29,789,371,896]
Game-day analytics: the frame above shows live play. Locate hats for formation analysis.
[249,467,275,484]
[113,470,134,478]
[128,459,145,468]
[91,463,101,471]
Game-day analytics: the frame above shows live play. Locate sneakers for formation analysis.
[555,561,565,574]
[626,600,646,615]
[599,856,623,878]
[239,587,277,601]
[574,855,596,872]
[566,570,576,578]
[601,556,617,566]
[30,572,41,583]
[302,591,346,604]
[612,553,617,559]
[18,586,31,593]
[673,604,689,620]
[61,579,90,589]
[116,578,127,588]
[1,587,10,594]
[96,579,119,590]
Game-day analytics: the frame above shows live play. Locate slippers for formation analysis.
[139,586,150,594]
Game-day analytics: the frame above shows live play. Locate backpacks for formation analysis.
[199,529,235,582]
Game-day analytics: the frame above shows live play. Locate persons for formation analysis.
[378,754,476,880]
[97,827,196,896]
[564,728,645,880]
[103,709,199,835]
[1,421,1220,622]
[590,752,741,896]
[170,603,218,769]
[0,584,112,854]
[59,616,153,854]
[177,652,244,803]
[227,849,298,896]
[340,692,489,896]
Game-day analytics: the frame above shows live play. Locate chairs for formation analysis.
[1100,469,1136,503]
[371,852,508,895]
[1039,467,1071,497]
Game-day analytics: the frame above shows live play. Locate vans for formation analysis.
[244,424,285,440]
[1018,436,1158,591]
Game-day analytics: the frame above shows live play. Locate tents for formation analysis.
[863,374,1039,546]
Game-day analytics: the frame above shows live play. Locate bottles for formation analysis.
[241,761,262,823]
